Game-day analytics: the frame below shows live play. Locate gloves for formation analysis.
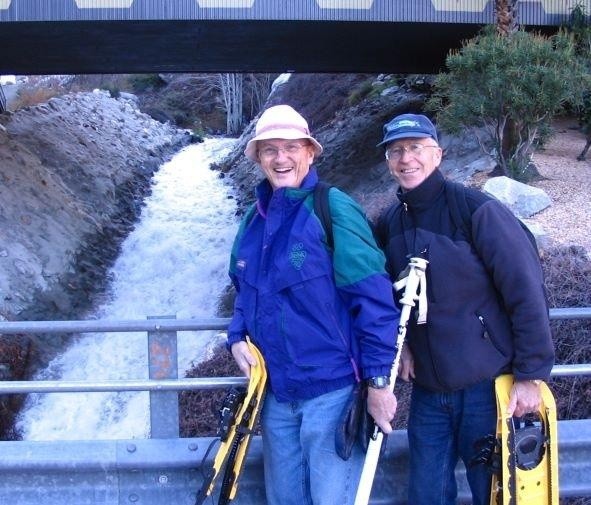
[333,381,362,462]
[357,385,387,459]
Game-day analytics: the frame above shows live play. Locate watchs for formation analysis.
[368,375,391,390]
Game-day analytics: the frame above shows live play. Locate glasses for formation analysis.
[385,143,437,160]
[259,141,313,155]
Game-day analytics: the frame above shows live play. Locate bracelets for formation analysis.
[526,378,544,387]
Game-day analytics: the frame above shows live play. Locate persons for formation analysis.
[366,110,556,505]
[225,104,403,505]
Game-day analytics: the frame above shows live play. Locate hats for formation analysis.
[375,113,439,150]
[243,104,324,164]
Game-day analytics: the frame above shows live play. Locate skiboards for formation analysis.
[195,335,267,505]
[473,374,559,505]
